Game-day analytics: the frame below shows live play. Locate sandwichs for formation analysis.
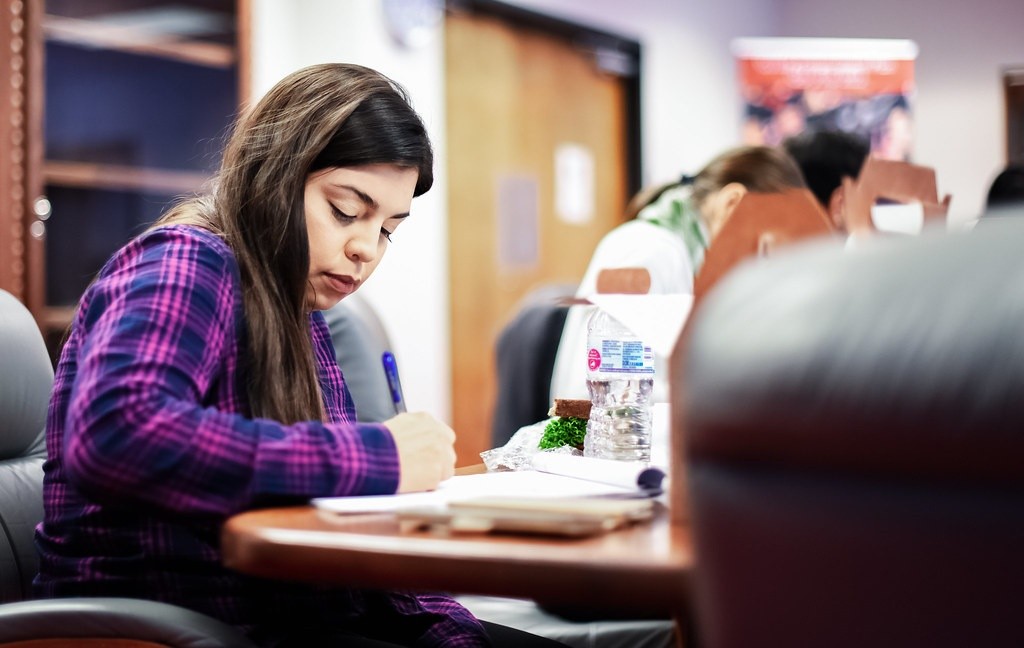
[537,399,592,450]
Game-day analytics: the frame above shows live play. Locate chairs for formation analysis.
[0,289,242,648]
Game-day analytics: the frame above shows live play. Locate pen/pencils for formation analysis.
[383,352,407,414]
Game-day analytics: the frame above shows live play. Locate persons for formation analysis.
[30,63,489,648]
[549,129,880,410]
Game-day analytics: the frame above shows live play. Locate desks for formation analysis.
[225,484,689,621]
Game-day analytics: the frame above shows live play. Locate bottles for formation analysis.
[583,307,656,470]
